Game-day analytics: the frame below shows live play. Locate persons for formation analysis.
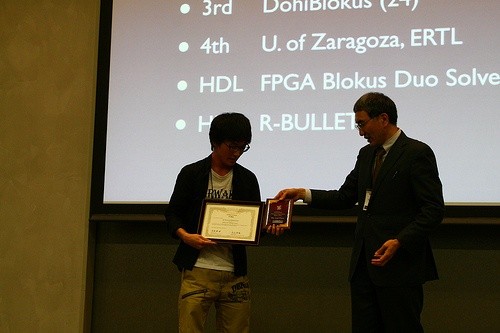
[275,92,445,333]
[166,113,284,333]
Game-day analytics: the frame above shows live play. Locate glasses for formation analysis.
[355,116,376,131]
[223,141,250,153]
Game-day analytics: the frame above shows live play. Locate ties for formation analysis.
[370,147,386,190]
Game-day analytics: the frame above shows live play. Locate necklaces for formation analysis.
[210,170,233,199]
